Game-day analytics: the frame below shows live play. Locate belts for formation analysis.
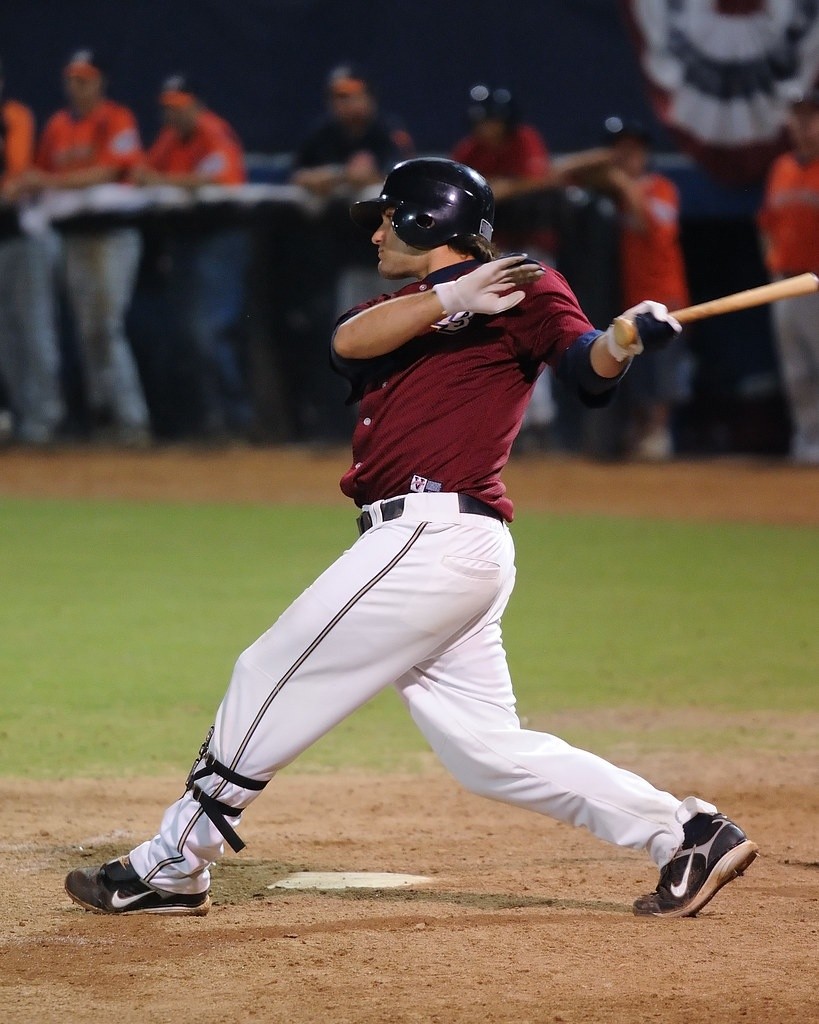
[354,492,503,536]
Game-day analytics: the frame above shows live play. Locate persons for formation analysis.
[0,47,260,446]
[288,64,690,462]
[64,156,759,920]
[758,90,819,467]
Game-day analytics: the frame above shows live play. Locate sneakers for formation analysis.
[63,853,213,919]
[631,810,760,919]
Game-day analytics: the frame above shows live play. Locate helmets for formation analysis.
[347,156,497,263]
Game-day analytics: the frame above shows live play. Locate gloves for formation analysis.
[433,252,547,318]
[605,300,684,361]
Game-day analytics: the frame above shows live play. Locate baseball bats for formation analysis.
[613,271,819,348]
